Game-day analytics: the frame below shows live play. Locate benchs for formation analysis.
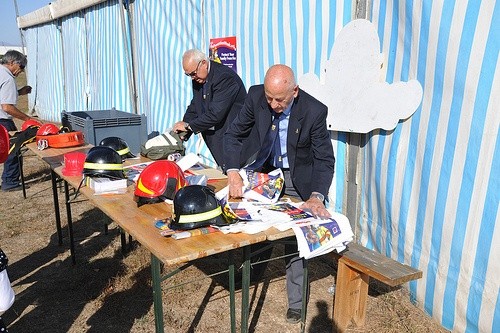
[326,242,423,333]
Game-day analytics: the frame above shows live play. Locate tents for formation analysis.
[16,0,500,333]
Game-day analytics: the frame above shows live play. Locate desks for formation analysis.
[8,130,308,333]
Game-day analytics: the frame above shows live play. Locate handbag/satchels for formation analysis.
[139,131,186,160]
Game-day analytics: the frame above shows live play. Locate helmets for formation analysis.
[21,119,43,131]
[82,146,124,179]
[36,123,61,136]
[133,159,188,203]
[168,185,229,230]
[99,136,136,157]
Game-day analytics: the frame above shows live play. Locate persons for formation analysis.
[0,49,43,193]
[0,248,15,333]
[222,65,336,323]
[173,50,260,177]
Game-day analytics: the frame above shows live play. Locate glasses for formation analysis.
[19,65,25,69]
[184,61,203,76]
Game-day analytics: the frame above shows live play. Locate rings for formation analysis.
[317,206,320,209]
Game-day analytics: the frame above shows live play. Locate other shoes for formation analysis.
[286,308,302,324]
[2,183,30,192]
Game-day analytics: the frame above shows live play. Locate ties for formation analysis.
[243,111,283,170]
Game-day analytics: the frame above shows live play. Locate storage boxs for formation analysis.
[61,107,148,154]
[82,173,127,193]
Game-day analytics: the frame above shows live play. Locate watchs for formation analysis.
[185,122,190,130]
[308,194,325,202]
